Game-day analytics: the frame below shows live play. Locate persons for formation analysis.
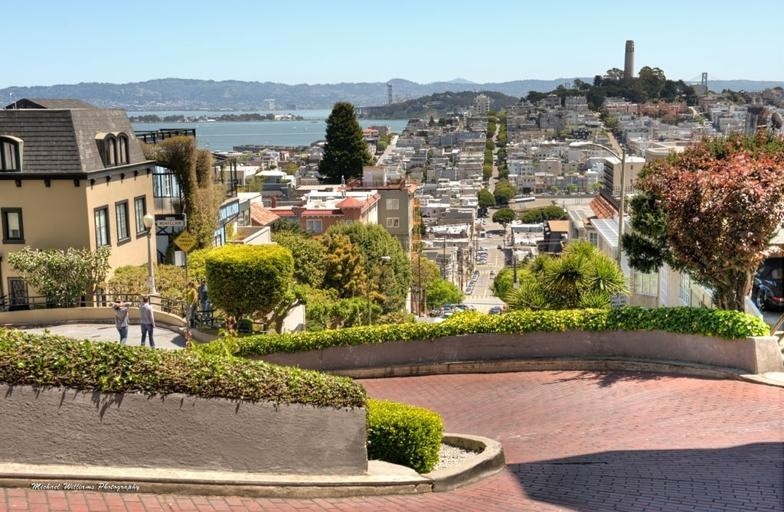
[139,298,156,350]
[112,296,133,348]
[187,280,199,330]
[197,279,211,314]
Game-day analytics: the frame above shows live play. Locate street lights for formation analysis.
[142,214,156,295]
[569,138,627,273]
